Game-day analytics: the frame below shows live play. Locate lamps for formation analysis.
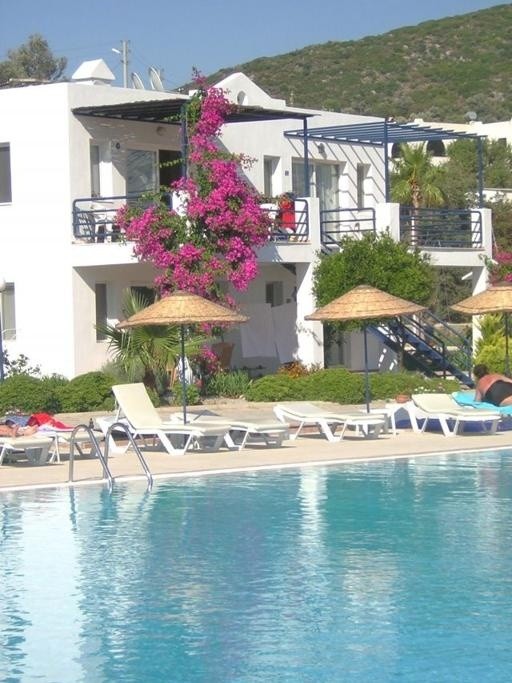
[317,143,326,153]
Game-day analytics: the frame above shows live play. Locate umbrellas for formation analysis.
[303,281,433,413]
[446,284,512,374]
[113,288,254,425]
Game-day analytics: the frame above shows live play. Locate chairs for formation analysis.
[256,202,279,239]
[195,338,234,374]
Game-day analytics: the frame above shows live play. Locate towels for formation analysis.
[452,390,512,418]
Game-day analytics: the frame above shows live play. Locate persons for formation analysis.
[469,362,512,412]
[1,419,40,439]
